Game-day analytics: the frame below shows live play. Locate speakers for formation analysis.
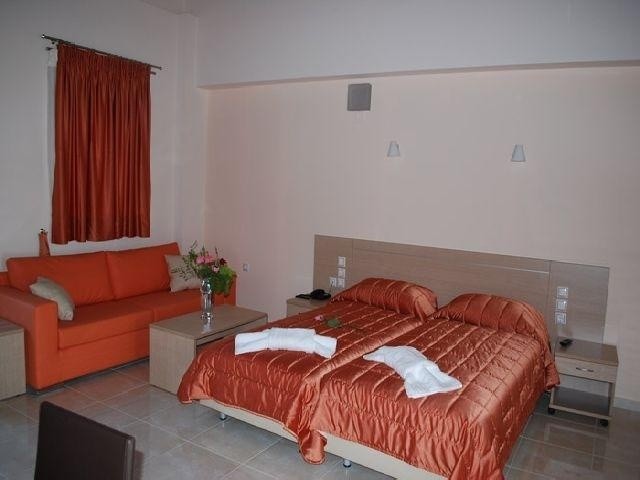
[348,83,371,112]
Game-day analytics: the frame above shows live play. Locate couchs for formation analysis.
[0,243,237,395]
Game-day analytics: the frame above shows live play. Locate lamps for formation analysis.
[511,145,525,162]
[387,141,400,157]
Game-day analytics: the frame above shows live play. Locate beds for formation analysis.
[310,319,560,480]
[176,302,436,465]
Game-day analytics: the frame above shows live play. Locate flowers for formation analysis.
[171,239,237,296]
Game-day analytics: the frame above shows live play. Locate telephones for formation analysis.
[310,289,331,300]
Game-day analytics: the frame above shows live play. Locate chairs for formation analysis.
[34,401,135,480]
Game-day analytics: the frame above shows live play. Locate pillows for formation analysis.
[425,293,549,347]
[29,276,75,321]
[164,254,201,292]
[329,277,438,322]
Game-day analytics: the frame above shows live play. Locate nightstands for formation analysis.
[286,297,329,317]
[547,336,619,427]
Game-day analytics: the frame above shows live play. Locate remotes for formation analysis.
[296,294,311,299]
[558,338,573,348]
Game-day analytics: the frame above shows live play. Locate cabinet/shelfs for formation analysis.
[0,318,27,400]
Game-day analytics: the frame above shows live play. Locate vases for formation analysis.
[200,280,213,332]
